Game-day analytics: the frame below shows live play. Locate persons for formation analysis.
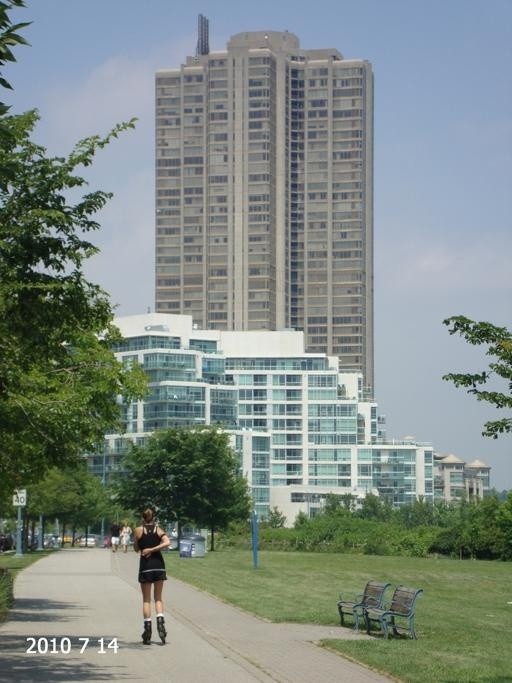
[133,507,171,641]
[120,521,133,552]
[110,519,122,552]
[171,528,177,538]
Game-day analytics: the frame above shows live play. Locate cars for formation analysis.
[0,530,136,553]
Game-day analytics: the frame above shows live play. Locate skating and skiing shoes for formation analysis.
[157,616,167,644]
[142,621,153,644]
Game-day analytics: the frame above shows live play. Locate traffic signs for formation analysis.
[13,487,25,505]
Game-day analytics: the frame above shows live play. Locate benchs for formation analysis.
[337,579,424,640]
